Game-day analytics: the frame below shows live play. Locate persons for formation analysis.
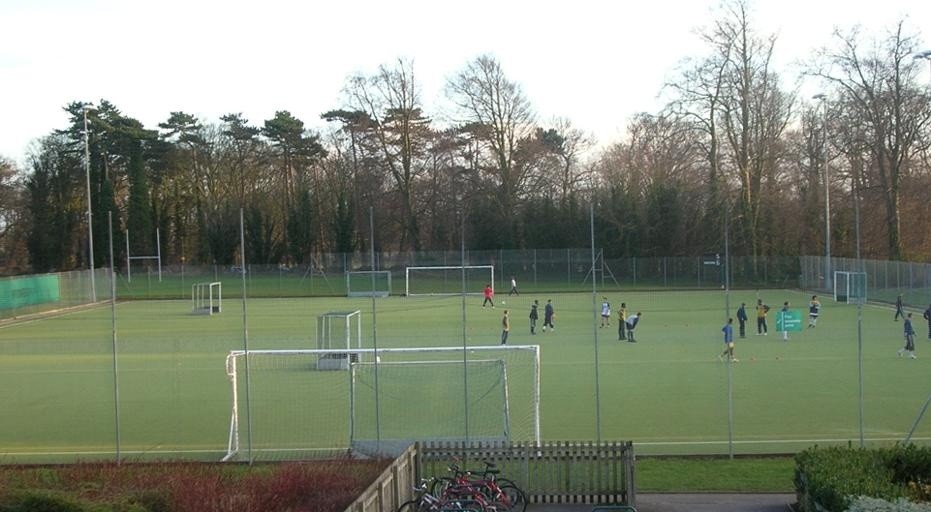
[483,284,495,309]
[529,299,540,333]
[501,309,512,346]
[719,318,740,362]
[893,293,907,322]
[618,301,628,341]
[781,299,792,340]
[755,299,771,335]
[600,297,612,329]
[625,312,643,343]
[508,276,519,296]
[810,295,821,329]
[541,298,557,332]
[898,313,919,360]
[737,301,749,339]
[924,305,931,341]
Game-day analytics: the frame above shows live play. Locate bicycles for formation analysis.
[395,454,530,512]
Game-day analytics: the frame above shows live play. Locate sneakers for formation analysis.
[898,350,917,359]
[599,323,610,328]
[719,354,739,362]
[756,332,767,336]
[619,337,637,342]
